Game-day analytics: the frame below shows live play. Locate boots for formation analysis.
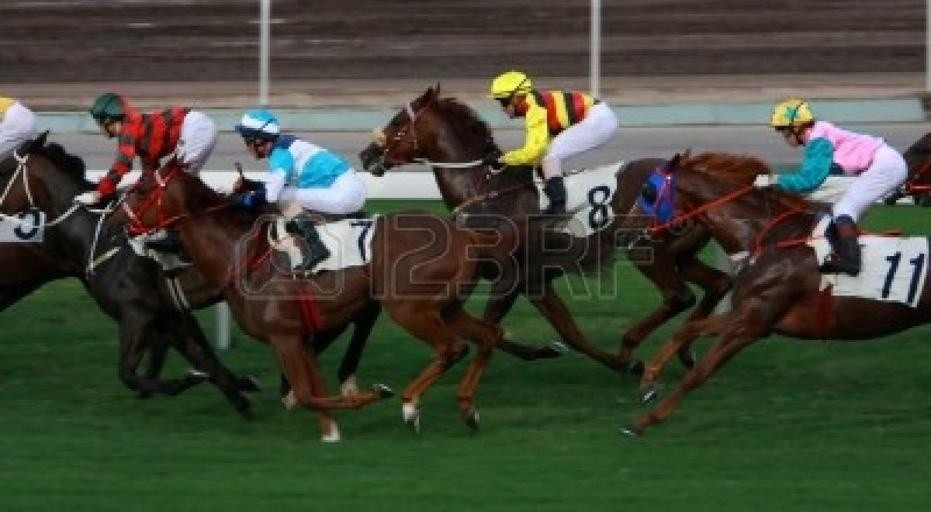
[819,215,863,277]
[286,212,331,273]
[536,176,568,221]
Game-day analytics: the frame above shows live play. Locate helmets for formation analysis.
[90,93,128,118]
[234,109,279,140]
[489,70,532,99]
[769,99,813,129]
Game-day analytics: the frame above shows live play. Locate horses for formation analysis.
[0,78,931,446]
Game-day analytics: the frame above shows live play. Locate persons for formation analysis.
[485,71,619,221]
[73,93,217,251]
[754,99,909,274]
[0,97,38,163]
[234,109,367,270]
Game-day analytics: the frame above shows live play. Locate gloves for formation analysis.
[753,174,777,188]
[489,155,504,169]
[236,179,266,198]
